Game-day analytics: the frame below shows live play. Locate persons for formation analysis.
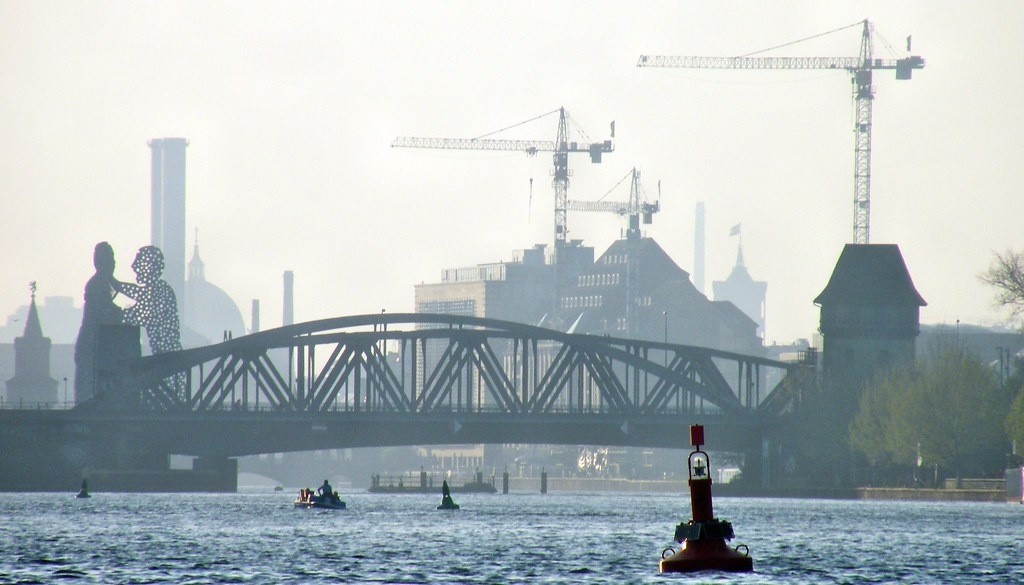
[318,479,340,500]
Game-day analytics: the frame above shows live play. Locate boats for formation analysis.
[293,489,346,510]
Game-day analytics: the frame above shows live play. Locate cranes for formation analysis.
[389,105,616,253]
[636,16,926,245]
[566,167,655,240]
